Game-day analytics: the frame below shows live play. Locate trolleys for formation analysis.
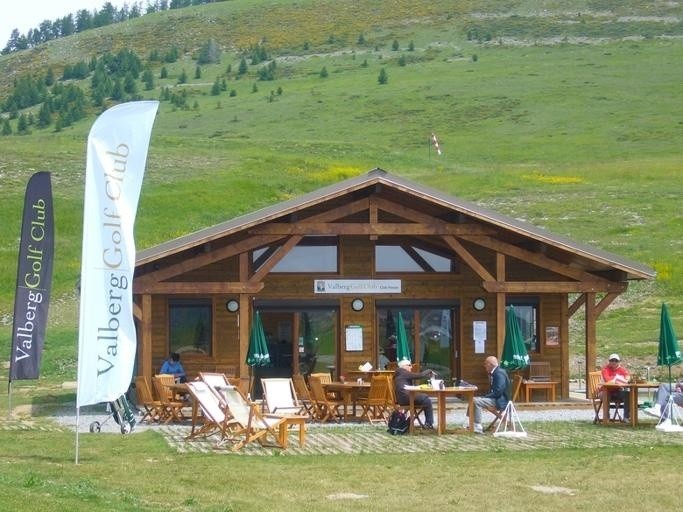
[89,382,136,434]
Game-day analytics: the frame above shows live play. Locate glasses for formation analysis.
[610,359,619,362]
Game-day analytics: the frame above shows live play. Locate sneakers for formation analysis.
[655,419,671,431]
[644,407,661,418]
[464,423,482,433]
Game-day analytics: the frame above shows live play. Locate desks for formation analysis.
[402,386,478,436]
[520,381,558,402]
[597,382,660,427]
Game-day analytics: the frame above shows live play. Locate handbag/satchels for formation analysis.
[388,411,409,435]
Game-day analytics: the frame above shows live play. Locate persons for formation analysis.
[393,359,436,430]
[463,355,513,434]
[157,352,188,402]
[382,334,398,366]
[595,352,632,425]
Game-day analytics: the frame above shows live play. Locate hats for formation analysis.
[609,354,619,360]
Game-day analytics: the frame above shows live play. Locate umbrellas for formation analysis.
[244,303,272,401]
[299,311,317,355]
[394,310,412,366]
[499,303,530,374]
[655,300,683,396]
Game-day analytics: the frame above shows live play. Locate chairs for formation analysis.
[530,363,552,400]
[134,370,428,452]
[588,371,623,423]
[483,374,523,433]
